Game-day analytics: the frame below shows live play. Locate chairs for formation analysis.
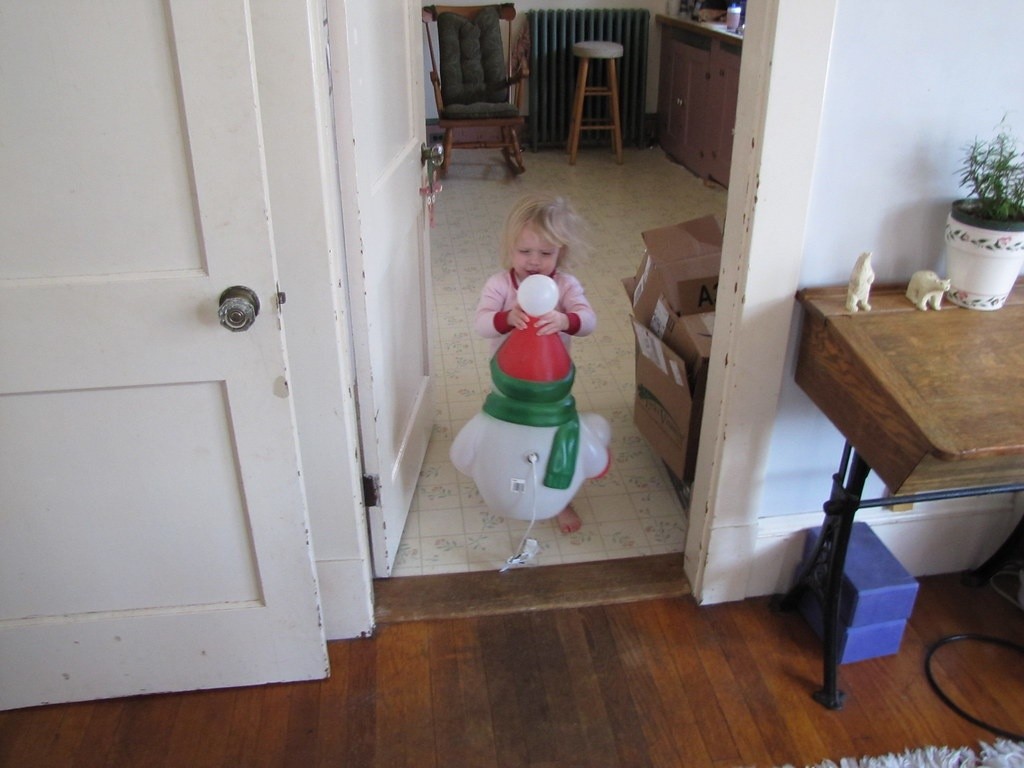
[422,2,536,173]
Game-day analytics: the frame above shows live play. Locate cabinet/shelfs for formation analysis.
[653,24,746,190]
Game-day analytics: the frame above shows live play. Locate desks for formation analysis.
[794,285,1023,715]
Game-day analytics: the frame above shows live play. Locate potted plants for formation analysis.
[939,113,1024,313]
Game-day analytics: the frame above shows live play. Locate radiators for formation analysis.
[522,7,652,150]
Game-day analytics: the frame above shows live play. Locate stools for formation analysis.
[565,41,626,167]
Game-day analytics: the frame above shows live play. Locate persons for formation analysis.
[472,188,597,534]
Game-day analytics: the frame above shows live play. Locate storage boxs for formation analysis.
[621,208,726,505]
[793,522,924,664]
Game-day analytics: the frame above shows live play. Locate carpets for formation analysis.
[779,733,1023,768]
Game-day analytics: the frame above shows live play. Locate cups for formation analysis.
[726,3,741,33]
